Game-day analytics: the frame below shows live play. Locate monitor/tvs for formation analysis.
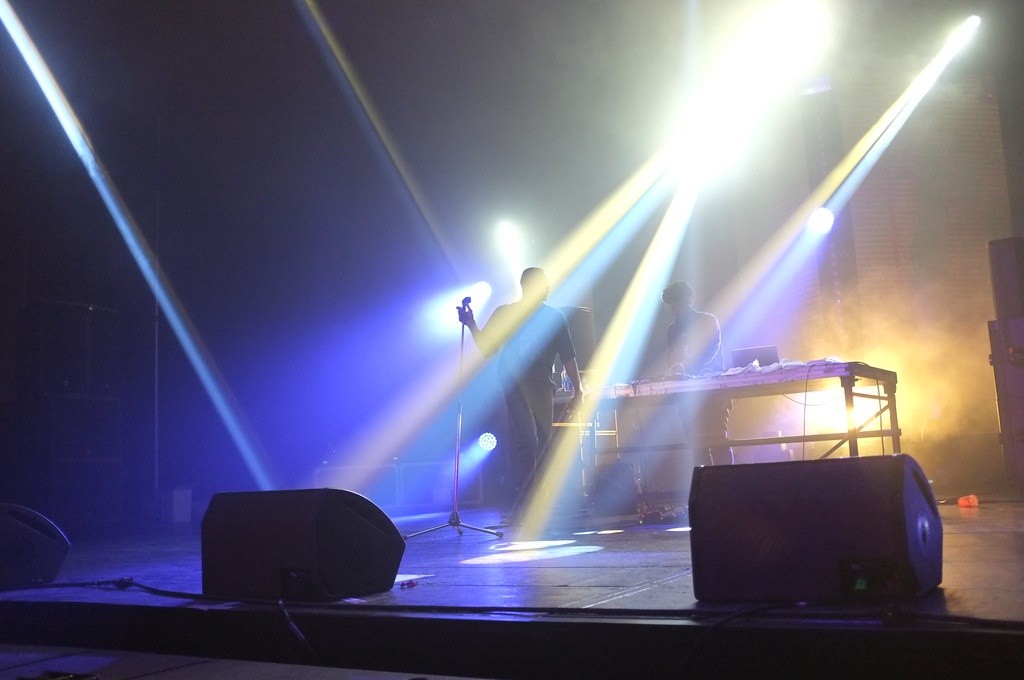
[731,345,780,369]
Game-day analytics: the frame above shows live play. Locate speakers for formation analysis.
[687,454,943,603]
[987,318,1024,496]
[201,488,406,605]
[985,236,1024,319]
[552,306,597,389]
[0,500,72,591]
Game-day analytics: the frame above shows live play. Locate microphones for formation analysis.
[462,297,471,306]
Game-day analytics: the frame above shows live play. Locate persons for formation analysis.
[662,280,734,466]
[456,268,583,511]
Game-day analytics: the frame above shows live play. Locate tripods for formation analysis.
[403,309,505,541]
